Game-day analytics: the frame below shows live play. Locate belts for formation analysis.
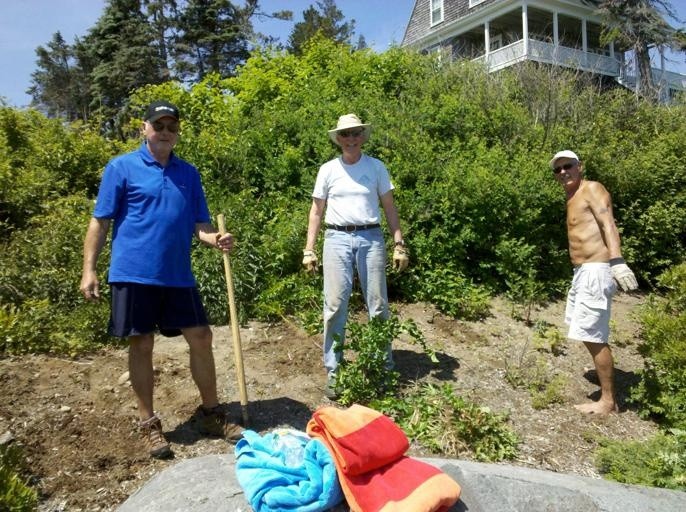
[326,223,380,232]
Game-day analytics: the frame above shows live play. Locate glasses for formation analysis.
[147,122,177,133]
[553,163,577,174]
[338,130,363,137]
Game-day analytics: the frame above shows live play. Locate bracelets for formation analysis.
[393,238,406,248]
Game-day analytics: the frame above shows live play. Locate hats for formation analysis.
[144,101,180,124]
[328,114,371,146]
[549,150,579,169]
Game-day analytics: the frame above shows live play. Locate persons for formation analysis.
[546,145,640,419]
[298,113,413,401]
[78,100,249,461]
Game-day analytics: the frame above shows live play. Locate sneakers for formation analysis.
[325,376,339,399]
[140,418,170,458]
[197,405,245,444]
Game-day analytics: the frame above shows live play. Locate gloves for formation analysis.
[609,257,639,291]
[302,249,319,275]
[391,245,410,274]
